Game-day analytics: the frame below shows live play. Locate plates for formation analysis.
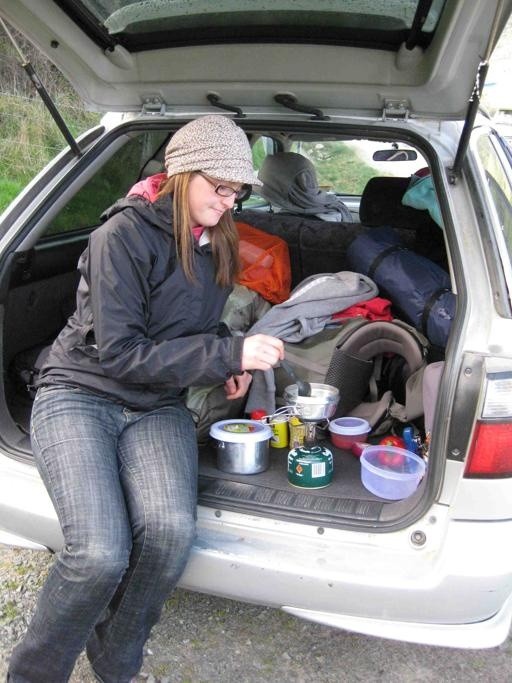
[208,418,273,444]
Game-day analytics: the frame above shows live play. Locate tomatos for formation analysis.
[377,435,406,466]
[351,441,372,459]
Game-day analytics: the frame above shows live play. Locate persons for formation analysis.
[4,113,285,682]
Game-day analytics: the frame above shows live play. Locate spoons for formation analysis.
[280,362,314,397]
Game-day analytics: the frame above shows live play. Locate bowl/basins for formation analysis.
[328,416,373,450]
[216,438,270,476]
[280,381,342,424]
[359,444,426,502]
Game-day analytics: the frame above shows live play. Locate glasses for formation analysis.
[195,172,250,200]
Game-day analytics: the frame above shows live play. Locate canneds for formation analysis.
[270,413,307,449]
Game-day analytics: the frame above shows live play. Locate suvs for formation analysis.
[0,0,512,650]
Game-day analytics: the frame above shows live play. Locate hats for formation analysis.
[164,114,268,186]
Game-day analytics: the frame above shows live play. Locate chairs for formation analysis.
[233,176,429,292]
